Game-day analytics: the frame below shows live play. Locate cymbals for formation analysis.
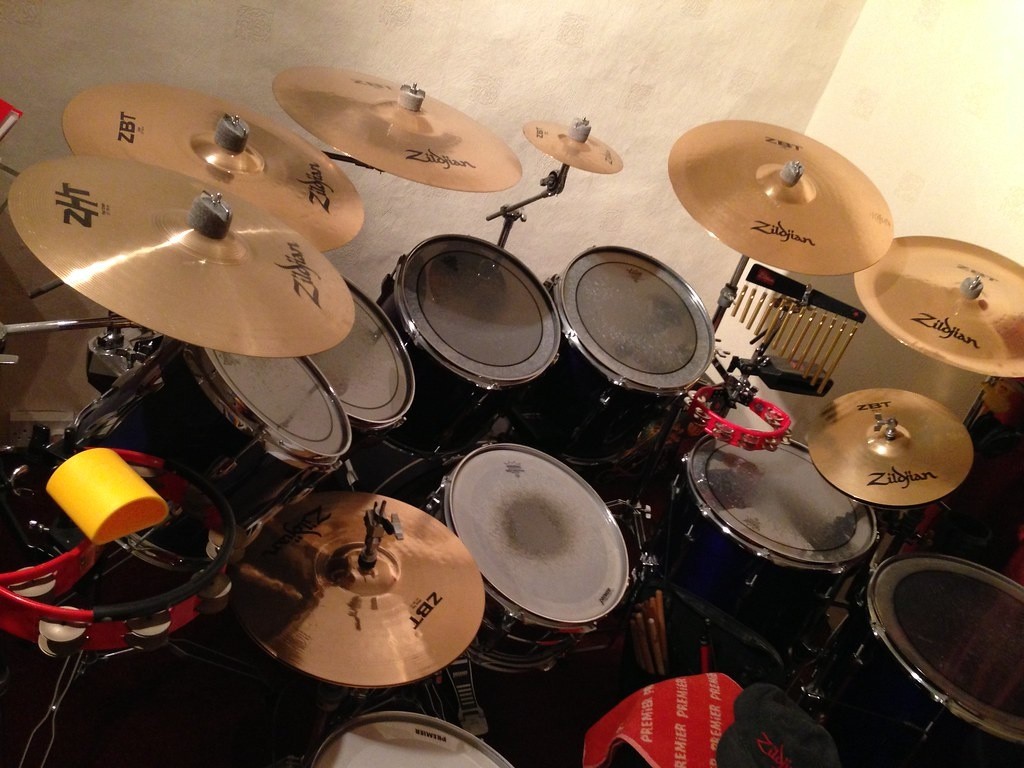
[63,83,364,252]
[273,65,523,192]
[668,120,894,276]
[11,467,244,657]
[809,388,973,506]
[235,493,486,688]
[854,236,1024,377]
[523,122,624,175]
[683,391,793,452]
[9,154,356,358]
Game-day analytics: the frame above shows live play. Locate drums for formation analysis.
[112,348,351,532]
[374,235,561,461]
[308,276,415,464]
[421,445,631,672]
[521,246,714,491]
[811,554,1024,767]
[648,433,880,654]
[308,711,514,768]
